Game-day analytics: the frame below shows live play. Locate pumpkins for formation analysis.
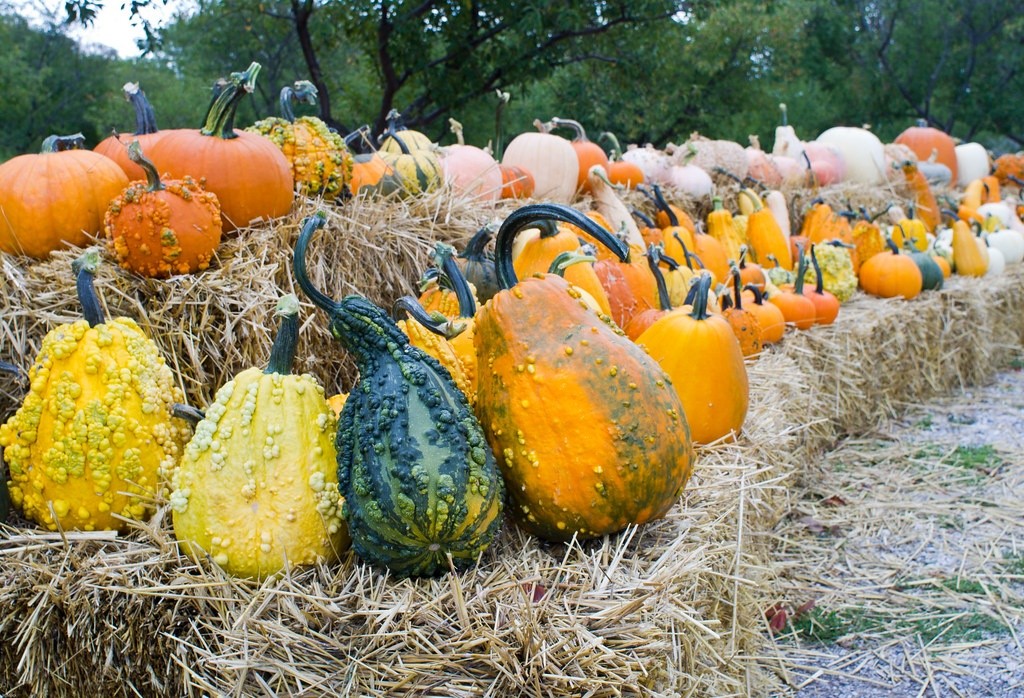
[0,62,1024,587]
